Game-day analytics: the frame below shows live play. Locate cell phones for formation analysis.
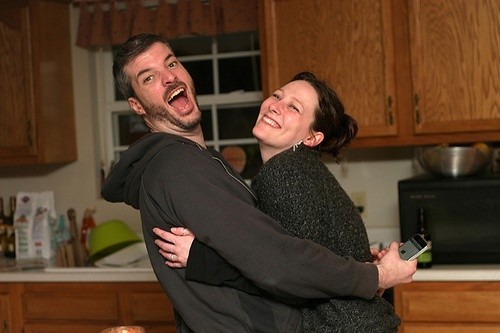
[399,234,428,261]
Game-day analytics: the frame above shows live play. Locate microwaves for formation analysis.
[397,173,500,264]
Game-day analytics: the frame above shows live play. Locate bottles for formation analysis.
[0,197,8,256]
[414,207,432,269]
[5,196,16,258]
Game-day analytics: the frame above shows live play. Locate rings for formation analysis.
[183,227,186,236]
[170,254,174,262]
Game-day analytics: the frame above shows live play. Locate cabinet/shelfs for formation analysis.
[0,281,500,333]
[257,0,500,149]
[0,0,78,167]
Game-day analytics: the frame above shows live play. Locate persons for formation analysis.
[153,71,401,333]
[101,33,417,333]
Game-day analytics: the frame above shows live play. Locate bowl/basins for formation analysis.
[88,220,143,262]
[415,147,494,181]
